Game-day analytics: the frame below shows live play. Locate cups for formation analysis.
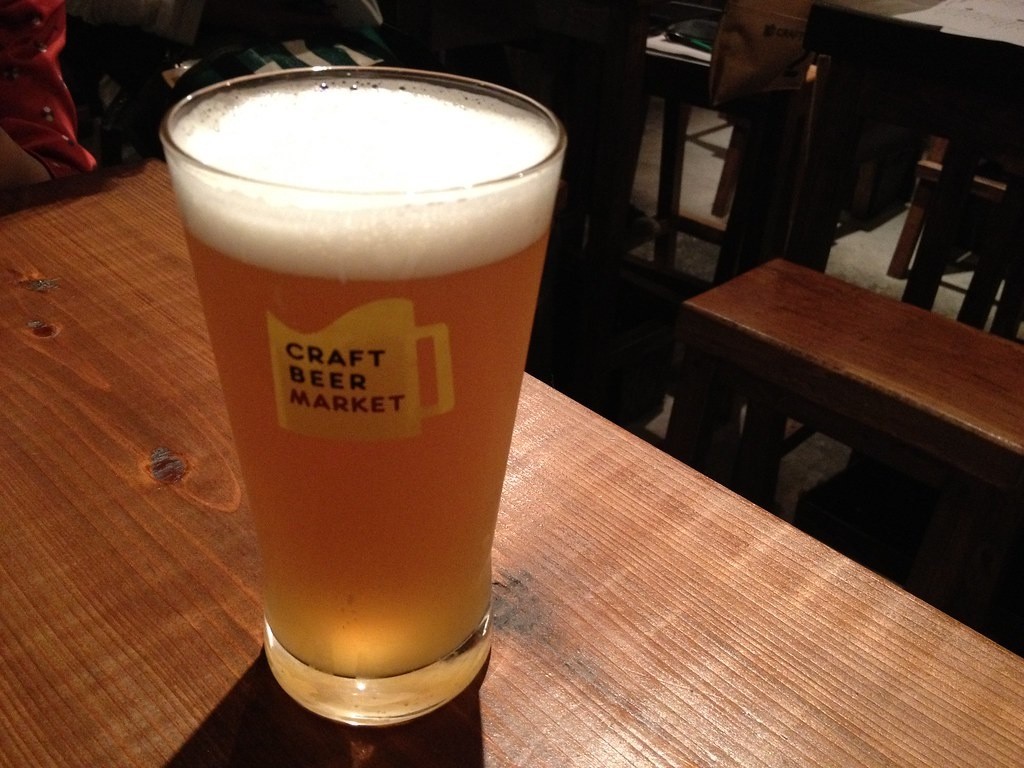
[161,66,568,727]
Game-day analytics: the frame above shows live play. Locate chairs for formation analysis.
[639,15,821,264]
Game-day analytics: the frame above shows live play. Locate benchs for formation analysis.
[670,259,1024,513]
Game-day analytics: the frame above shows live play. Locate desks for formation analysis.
[0,135,1024,768]
[640,0,1024,264]
